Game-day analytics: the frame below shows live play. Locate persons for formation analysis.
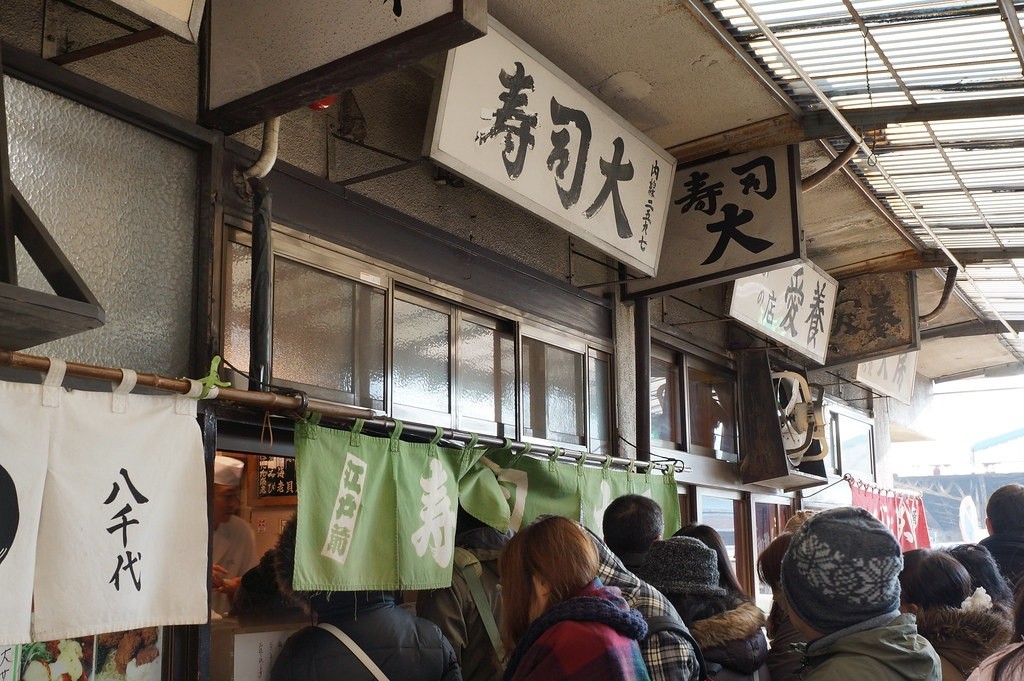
[228,550,299,615]
[416,537,503,681]
[779,506,942,681]
[979,483,1024,574]
[756,509,810,681]
[271,510,462,681]
[898,542,1024,681]
[212,455,257,615]
[494,514,699,681]
[603,494,767,681]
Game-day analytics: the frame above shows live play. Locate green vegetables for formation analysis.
[21,641,53,674]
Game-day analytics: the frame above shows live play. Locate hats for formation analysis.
[783,509,816,533]
[214,456,245,486]
[780,507,904,631]
[635,536,726,597]
